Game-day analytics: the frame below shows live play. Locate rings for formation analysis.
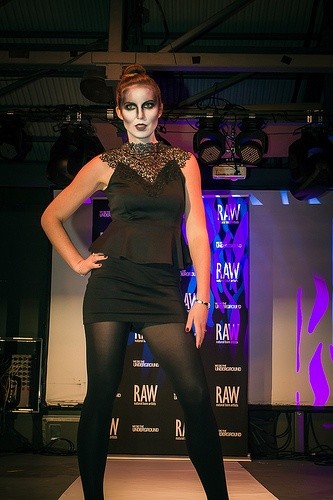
[204,330,207,332]
[93,253,95,257]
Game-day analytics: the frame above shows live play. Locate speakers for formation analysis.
[0,337,43,415]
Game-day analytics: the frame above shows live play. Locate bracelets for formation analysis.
[196,300,208,307]
[79,260,92,278]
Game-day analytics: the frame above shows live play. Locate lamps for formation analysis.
[46,128,83,186]
[288,126,333,201]
[236,118,268,165]
[193,118,227,165]
[0,117,31,163]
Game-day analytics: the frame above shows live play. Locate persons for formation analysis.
[41,64,230,500]
